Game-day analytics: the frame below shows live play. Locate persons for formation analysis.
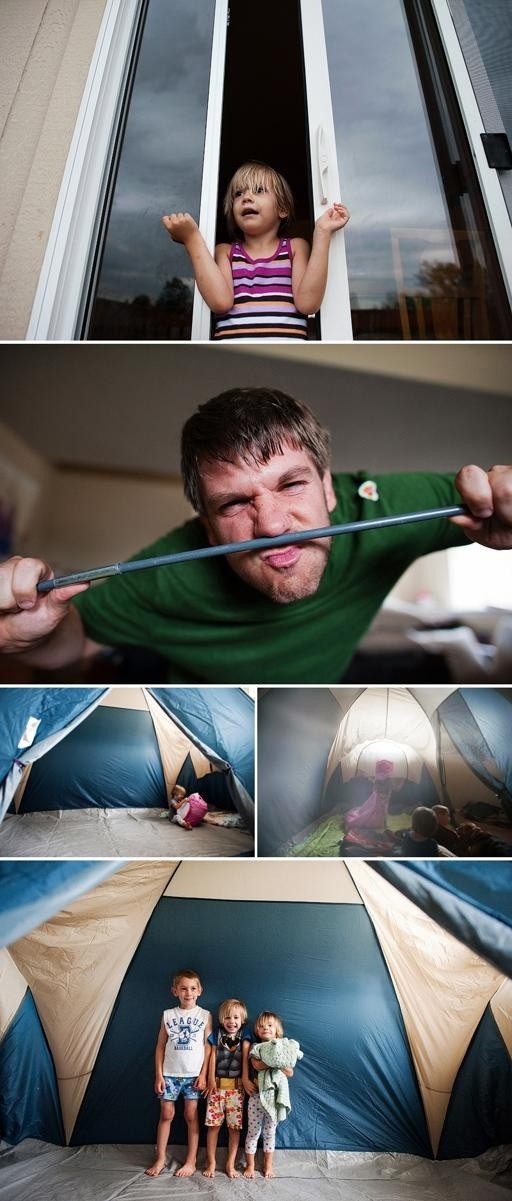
[372,761,406,827]
[161,162,352,344]
[200,998,257,1178]
[168,784,195,830]
[432,802,494,857]
[399,806,441,858]
[0,382,511,685]
[143,967,216,1178]
[241,1011,295,1180]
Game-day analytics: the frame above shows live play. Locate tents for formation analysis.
[0,859,510,1201]
[257,687,510,858]
[2,688,255,855]
